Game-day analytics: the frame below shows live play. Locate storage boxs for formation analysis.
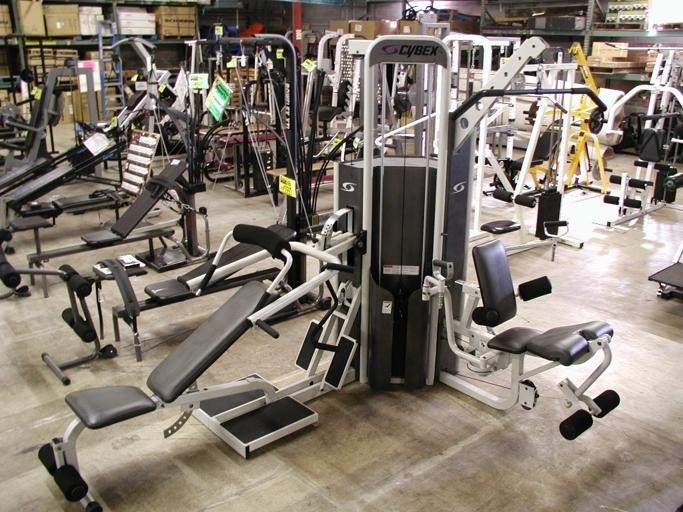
[103,51,114,79]
[58,92,75,124]
[13,0,46,35]
[78,60,101,93]
[350,21,382,41]
[593,42,628,60]
[153,6,195,36]
[72,88,91,124]
[400,21,422,35]
[329,20,356,46]
[42,5,80,35]
[86,52,100,60]
[79,7,104,35]
[97,88,117,120]
[0,5,12,35]
